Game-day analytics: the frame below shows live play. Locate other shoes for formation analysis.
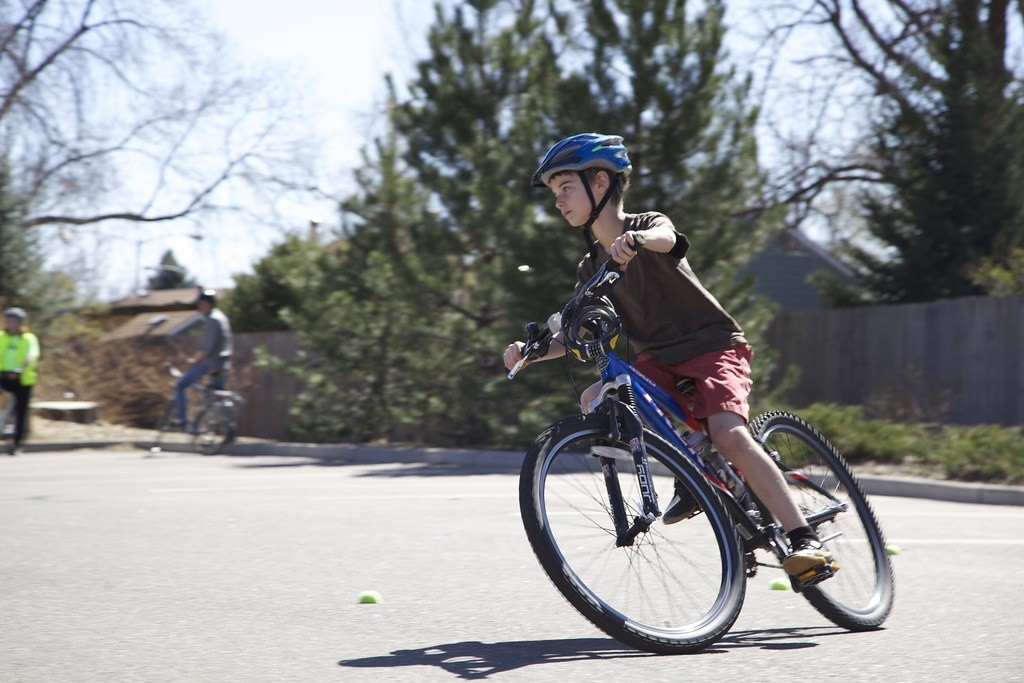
[170,419,186,426]
[9,444,22,456]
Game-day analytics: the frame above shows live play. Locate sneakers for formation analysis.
[783,538,839,579]
[662,475,698,524]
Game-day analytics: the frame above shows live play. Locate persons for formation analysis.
[170,289,236,442]
[502,132,840,585]
[0,307,41,455]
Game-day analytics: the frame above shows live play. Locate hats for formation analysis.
[191,291,215,304]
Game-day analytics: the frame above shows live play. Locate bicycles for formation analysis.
[157,354,246,455]
[504,234,894,653]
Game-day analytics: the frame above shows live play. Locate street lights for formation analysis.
[92,231,203,294]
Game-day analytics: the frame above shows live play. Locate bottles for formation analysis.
[681,430,746,500]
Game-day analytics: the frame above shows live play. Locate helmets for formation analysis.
[4,307,25,318]
[530,132,633,188]
